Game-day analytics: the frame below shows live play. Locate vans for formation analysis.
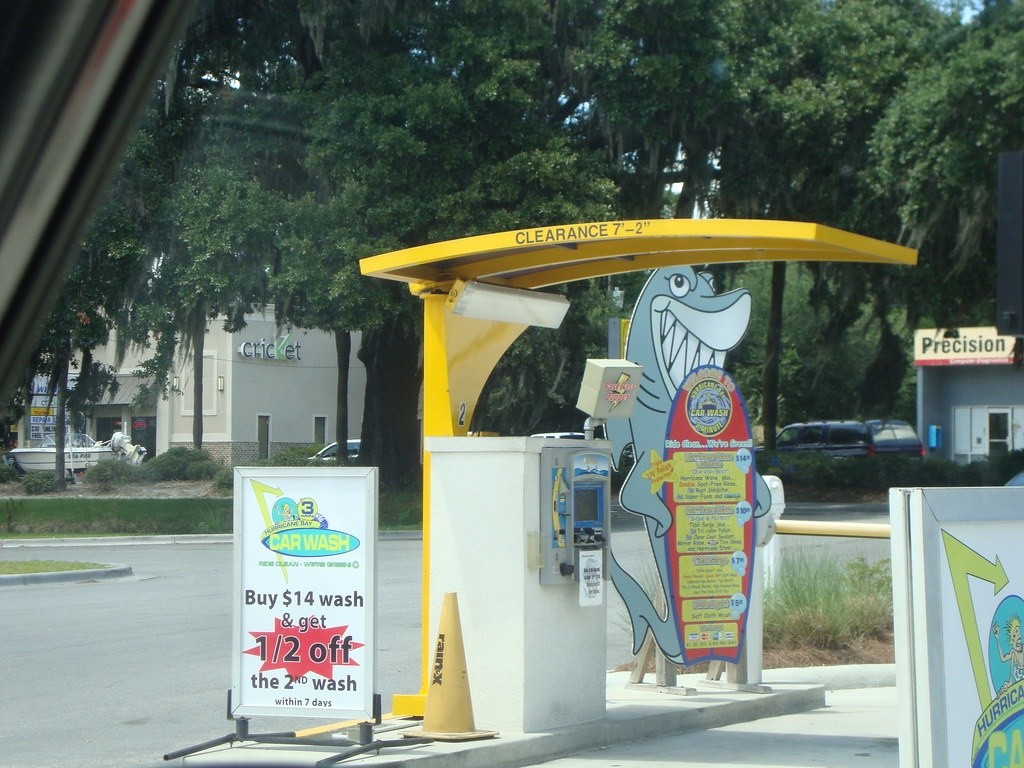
[754,420,925,461]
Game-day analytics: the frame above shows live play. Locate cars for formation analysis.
[307,439,362,462]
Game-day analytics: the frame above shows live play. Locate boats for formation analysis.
[9,411,148,485]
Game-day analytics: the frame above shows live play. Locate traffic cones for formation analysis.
[402,593,500,742]
